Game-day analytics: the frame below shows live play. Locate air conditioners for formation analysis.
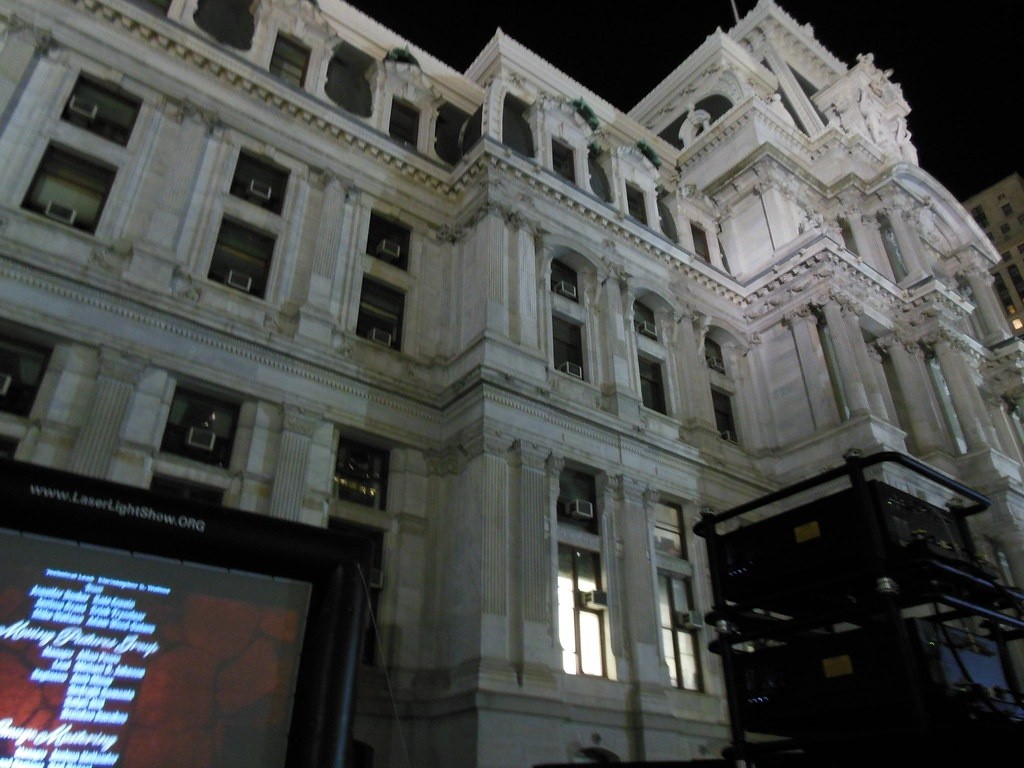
[561,361,583,378]
[248,179,272,201]
[677,610,703,628]
[70,97,96,118]
[572,501,594,517]
[553,280,577,298]
[582,591,607,609]
[43,199,79,224]
[370,328,393,346]
[376,238,402,260]
[708,356,725,371]
[721,430,739,445]
[186,427,215,451]
[638,320,657,336]
[227,270,252,293]
